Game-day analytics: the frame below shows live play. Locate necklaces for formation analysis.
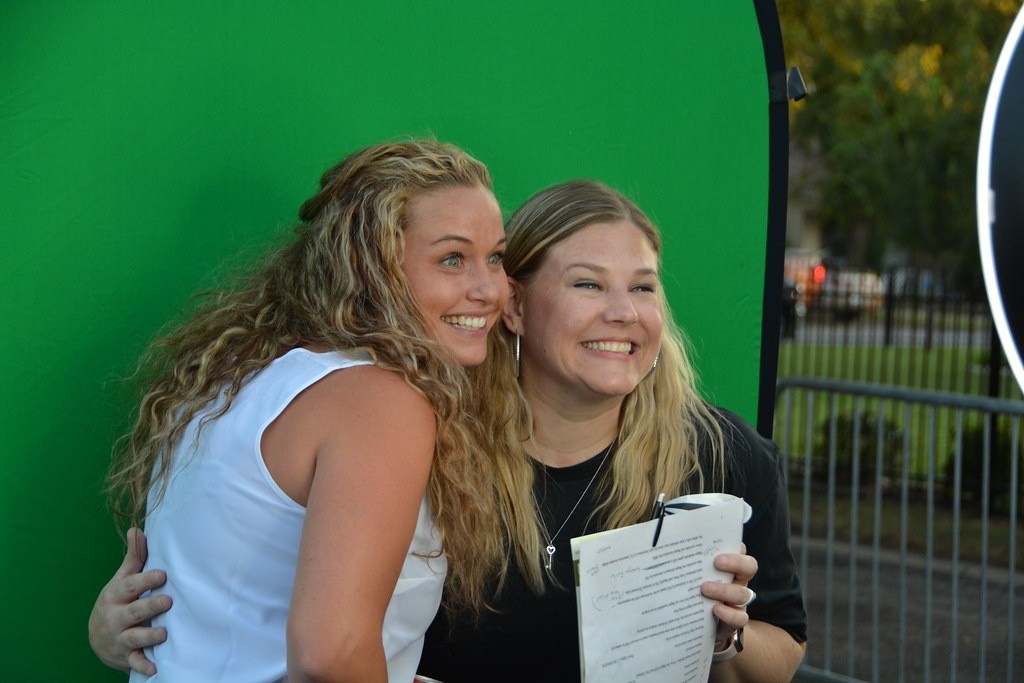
[531,433,620,570]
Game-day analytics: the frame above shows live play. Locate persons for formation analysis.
[90,140,807,683]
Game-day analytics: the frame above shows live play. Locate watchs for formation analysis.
[711,626,744,663]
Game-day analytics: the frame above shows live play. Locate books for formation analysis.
[570,493,753,683]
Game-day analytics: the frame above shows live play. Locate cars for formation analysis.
[816,269,885,322]
[782,247,827,336]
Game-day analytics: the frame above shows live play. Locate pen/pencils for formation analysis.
[733,625,745,653]
[650,491,666,547]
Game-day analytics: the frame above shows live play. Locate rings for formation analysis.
[733,585,757,608]
